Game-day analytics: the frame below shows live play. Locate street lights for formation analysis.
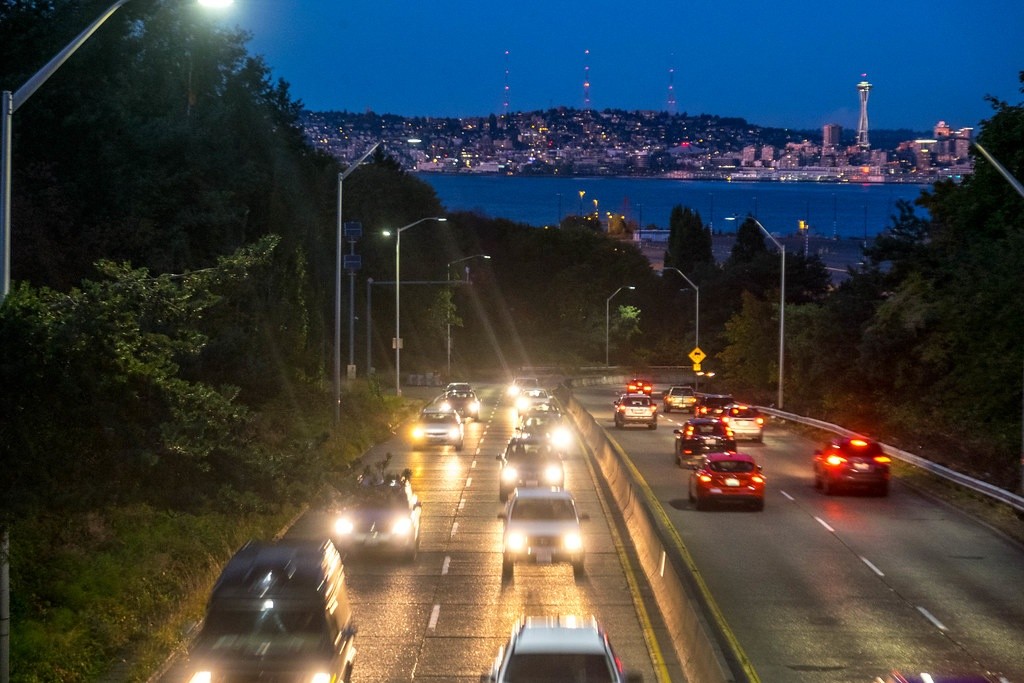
[332,137,422,425]
[0,0,237,313]
[725,217,785,411]
[606,284,636,369]
[656,266,699,392]
[395,216,448,396]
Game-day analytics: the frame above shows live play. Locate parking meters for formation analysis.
[446,254,491,382]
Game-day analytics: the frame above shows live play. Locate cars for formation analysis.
[687,451,766,511]
[510,375,568,460]
[332,481,422,563]
[626,379,653,396]
[812,436,891,497]
[496,438,566,504]
[442,380,483,424]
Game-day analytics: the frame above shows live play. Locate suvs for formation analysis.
[480,614,644,683]
[497,486,591,581]
[693,394,736,422]
[664,385,696,414]
[720,404,766,443]
[673,417,737,468]
[413,409,466,451]
[614,393,658,430]
[175,538,356,683]
